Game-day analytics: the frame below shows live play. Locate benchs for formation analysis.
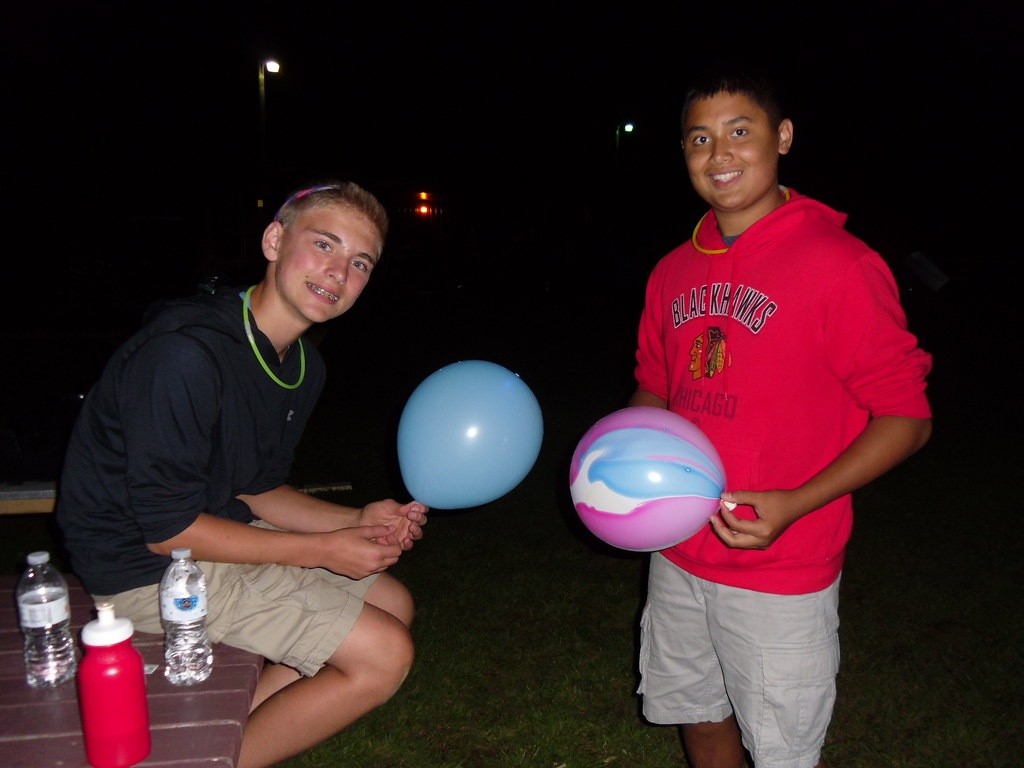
[0,572,266,768]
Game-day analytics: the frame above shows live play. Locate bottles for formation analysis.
[76,600,155,767]
[157,547,214,687]
[20,551,75,689]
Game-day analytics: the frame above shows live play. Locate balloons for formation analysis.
[568,405,736,555]
[398,359,545,508]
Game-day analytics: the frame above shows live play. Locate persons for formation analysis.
[53,179,415,764]
[619,76,932,766]
